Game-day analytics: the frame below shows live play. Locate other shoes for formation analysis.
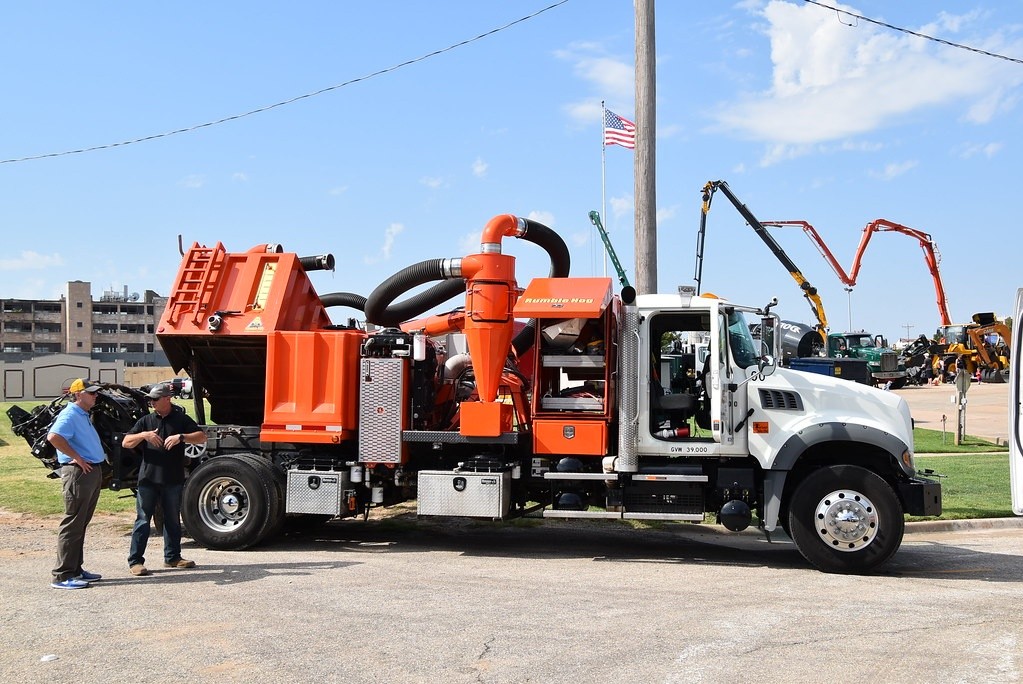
[163,559,196,568]
[127,562,147,576]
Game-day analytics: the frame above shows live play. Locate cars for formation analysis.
[170,378,182,396]
[180,378,194,399]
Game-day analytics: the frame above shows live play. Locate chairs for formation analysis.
[650,351,697,421]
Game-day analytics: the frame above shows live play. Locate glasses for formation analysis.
[88,390,98,395]
[147,397,159,401]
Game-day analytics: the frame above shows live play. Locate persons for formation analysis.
[122,384,207,576]
[936,357,944,382]
[920,353,932,386]
[46,379,105,590]
[976,368,982,385]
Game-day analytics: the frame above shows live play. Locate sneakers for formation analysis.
[71,570,102,581]
[51,577,90,589]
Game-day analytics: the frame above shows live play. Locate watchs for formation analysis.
[179,434,184,442]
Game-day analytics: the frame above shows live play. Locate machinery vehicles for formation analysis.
[744,218,1008,383]
[682,178,909,389]
[965,311,1013,382]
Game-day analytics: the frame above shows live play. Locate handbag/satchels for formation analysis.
[136,448,172,488]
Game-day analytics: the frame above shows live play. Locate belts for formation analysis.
[59,463,79,467]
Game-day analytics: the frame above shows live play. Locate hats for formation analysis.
[145,384,171,398]
[69,378,103,394]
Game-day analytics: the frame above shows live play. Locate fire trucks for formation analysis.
[6,213,942,575]
